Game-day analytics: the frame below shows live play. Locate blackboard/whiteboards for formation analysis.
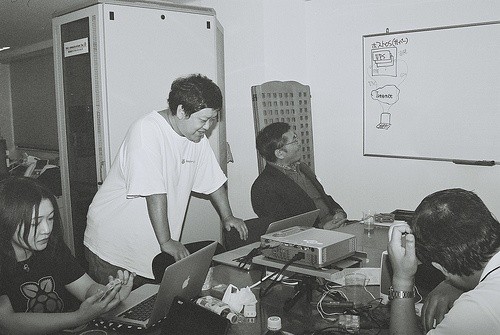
[362,21,500,163]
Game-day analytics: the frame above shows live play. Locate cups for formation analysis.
[338,314,360,335]
[80,330,107,335]
[363,208,375,230]
[344,273,366,306]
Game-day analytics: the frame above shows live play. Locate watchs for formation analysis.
[388,285,416,301]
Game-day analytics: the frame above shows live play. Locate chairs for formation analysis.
[223,218,276,251]
[152,241,225,284]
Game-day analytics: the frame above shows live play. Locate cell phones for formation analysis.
[99,278,120,303]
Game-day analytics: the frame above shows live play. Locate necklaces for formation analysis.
[17,259,30,272]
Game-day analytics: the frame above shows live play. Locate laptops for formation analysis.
[160,295,230,335]
[97,240,217,329]
[212,209,320,270]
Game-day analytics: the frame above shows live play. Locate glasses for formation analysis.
[281,133,298,147]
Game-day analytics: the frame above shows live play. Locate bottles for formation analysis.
[195,295,237,324]
[265,316,285,335]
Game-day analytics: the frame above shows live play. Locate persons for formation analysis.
[82,74,249,290]
[386,188,500,335]
[250,122,360,230]
[0,175,136,335]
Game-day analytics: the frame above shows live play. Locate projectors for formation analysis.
[260,225,357,268]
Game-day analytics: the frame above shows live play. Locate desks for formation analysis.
[202,222,392,335]
